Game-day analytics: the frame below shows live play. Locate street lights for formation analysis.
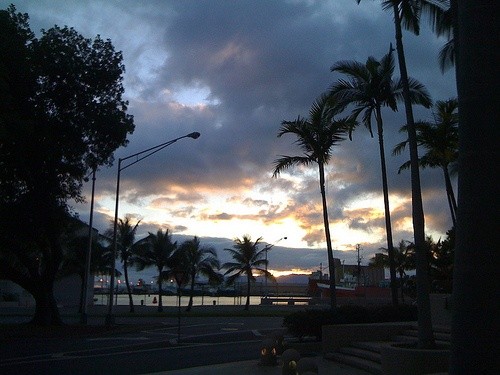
[264,237,288,299]
[103,131,201,325]
[80,166,97,325]
